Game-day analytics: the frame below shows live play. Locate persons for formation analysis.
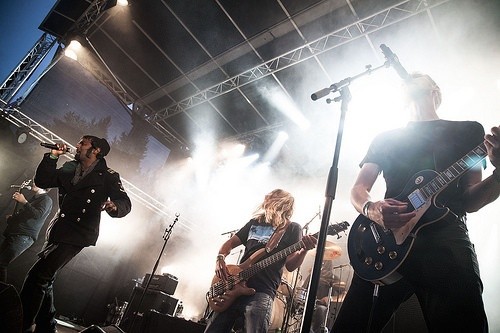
[204,190,318,333]
[0,177,53,284]
[20,135,132,333]
[330,73,500,333]
[302,260,346,333]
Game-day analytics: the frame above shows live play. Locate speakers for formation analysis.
[0,283,24,333]
[119,287,179,333]
[79,324,127,333]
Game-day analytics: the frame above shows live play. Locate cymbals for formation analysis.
[318,277,348,290]
[307,240,344,261]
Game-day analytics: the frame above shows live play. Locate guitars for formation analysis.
[1,178,32,240]
[347,133,489,289]
[208,220,350,314]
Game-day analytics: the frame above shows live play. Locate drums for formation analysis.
[267,280,295,333]
[277,265,298,296]
[291,286,318,311]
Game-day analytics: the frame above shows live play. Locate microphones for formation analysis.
[39,143,71,152]
[380,43,402,69]
[10,185,31,190]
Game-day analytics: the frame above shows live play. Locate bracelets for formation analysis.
[493,170,500,183]
[217,254,224,260]
[50,154,57,159]
[363,201,373,216]
